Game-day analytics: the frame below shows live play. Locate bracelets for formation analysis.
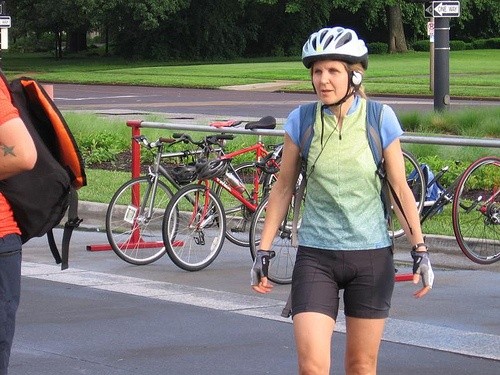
[412,243,428,251]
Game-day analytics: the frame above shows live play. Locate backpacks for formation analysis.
[0,70,87,270]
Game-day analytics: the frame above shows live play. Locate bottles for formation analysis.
[225,172,246,194]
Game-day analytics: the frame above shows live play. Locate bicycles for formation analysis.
[105,116,500,286]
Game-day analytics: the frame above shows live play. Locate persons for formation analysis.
[251,27,433,374]
[0,66,38,375]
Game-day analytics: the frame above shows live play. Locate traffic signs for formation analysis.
[423,1,461,18]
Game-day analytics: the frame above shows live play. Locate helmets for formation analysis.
[301,26,369,69]
[195,158,232,179]
[169,162,197,185]
